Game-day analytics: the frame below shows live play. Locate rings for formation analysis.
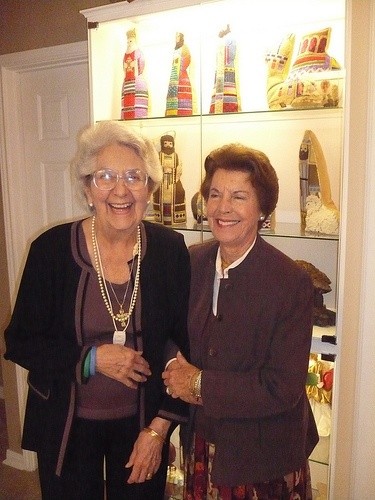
[165,386,171,395]
[147,473,153,478]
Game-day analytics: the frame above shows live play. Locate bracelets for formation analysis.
[83,346,95,377]
[195,369,203,400]
[143,426,165,444]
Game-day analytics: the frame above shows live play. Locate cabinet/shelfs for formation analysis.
[79,0,375,500]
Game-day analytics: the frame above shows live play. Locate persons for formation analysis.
[4,121,191,500]
[162,143,320,500]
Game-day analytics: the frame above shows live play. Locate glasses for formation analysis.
[85,168,149,190]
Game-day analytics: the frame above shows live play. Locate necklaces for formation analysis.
[92,216,141,346]
